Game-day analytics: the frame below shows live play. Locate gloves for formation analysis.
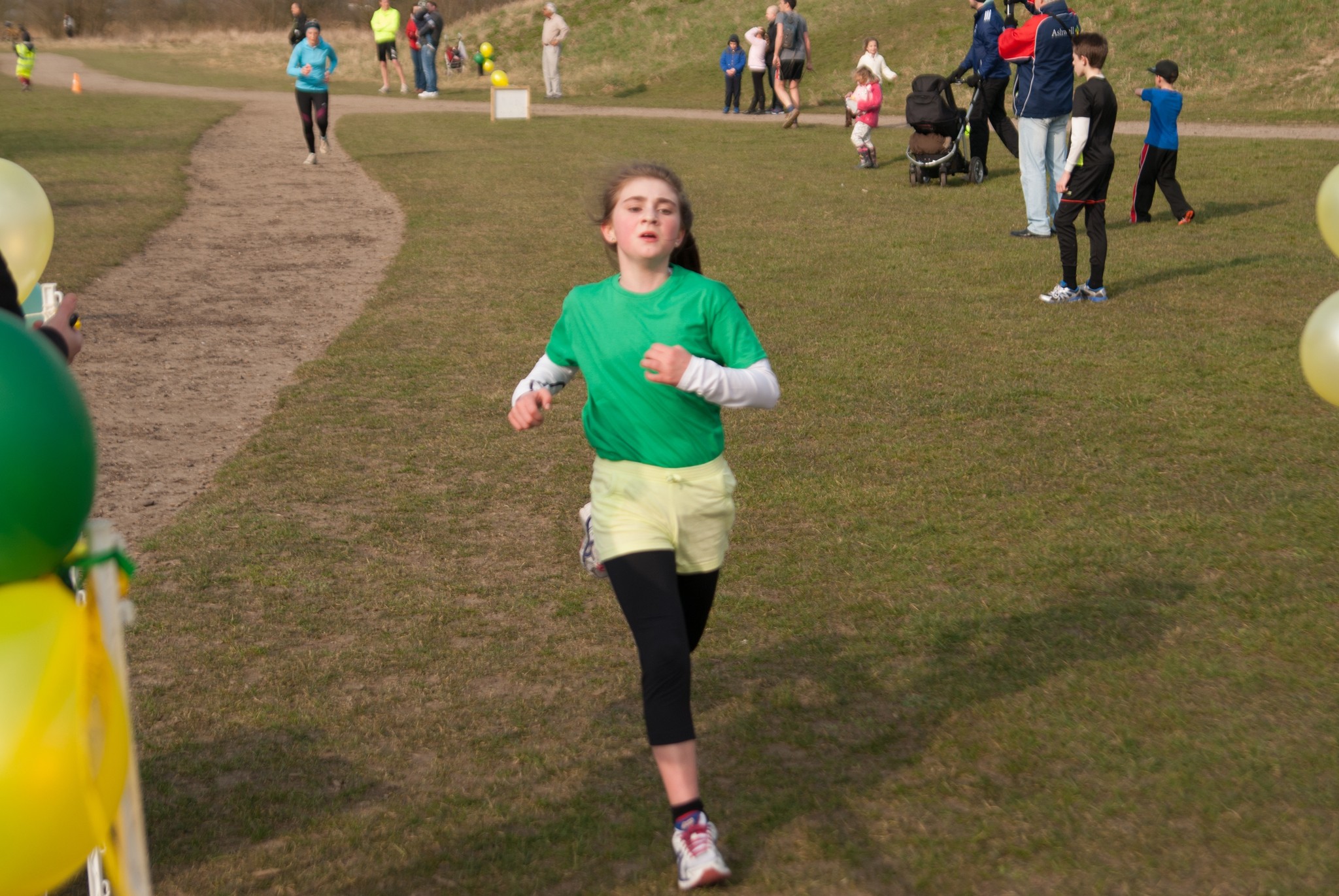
[947,66,966,83]
[1004,0,1025,5]
[1004,13,1018,27]
[966,72,981,87]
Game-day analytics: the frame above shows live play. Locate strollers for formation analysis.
[443,32,466,74]
[901,72,985,187]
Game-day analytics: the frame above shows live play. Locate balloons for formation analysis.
[1298,289,1339,408]
[474,41,510,87]
[1312,162,1338,263]
[1,153,136,896]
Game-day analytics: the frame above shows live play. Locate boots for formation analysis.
[854,143,879,169]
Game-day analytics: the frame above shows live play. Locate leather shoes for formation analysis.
[1011,227,1051,238]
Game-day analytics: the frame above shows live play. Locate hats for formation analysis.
[304,17,320,34]
[1146,59,1179,78]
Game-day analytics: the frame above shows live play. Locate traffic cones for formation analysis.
[71,72,81,94]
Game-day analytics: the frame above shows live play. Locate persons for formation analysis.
[720,1,813,130]
[949,0,1021,176]
[998,0,1081,240]
[844,64,883,169]
[1134,59,1195,226]
[288,1,306,51]
[413,1,444,99]
[507,162,781,892]
[286,18,338,166]
[404,3,427,95]
[855,36,898,90]
[3,18,35,89]
[369,0,409,95]
[412,0,436,51]
[540,2,569,98]
[1038,33,1119,305]
[63,12,76,37]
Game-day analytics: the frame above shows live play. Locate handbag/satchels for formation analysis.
[780,10,800,50]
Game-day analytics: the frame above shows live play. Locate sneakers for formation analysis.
[722,105,799,128]
[1178,210,1194,225]
[671,810,731,889]
[319,135,329,154]
[1077,278,1108,302]
[579,502,608,578]
[303,153,317,165]
[1038,280,1082,303]
